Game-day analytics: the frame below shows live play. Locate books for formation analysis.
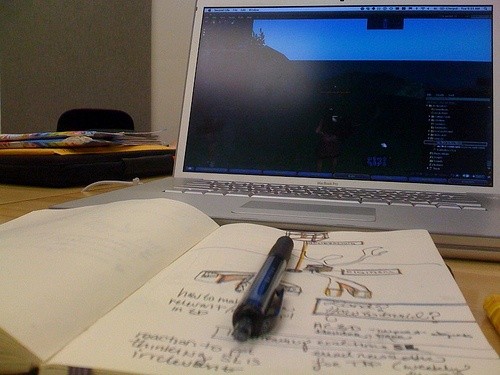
[0,198,500,375]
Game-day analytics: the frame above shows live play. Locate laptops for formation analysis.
[49,0,500,260]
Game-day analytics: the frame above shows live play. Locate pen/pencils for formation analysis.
[228,232,299,345]
